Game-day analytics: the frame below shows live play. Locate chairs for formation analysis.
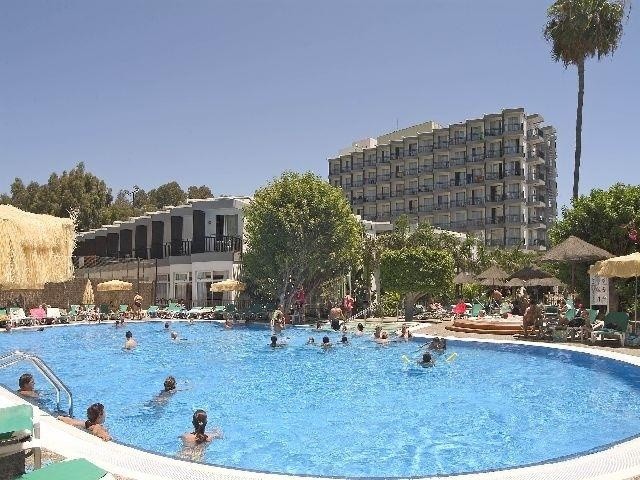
[1,404,108,480]
[548,308,629,347]
[460,304,484,319]
[0,302,227,327]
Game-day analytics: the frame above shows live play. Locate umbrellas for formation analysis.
[209,277,246,306]
[97,279,134,309]
[451,234,640,341]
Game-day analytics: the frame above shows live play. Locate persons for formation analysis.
[179,409,224,460]
[57,402,112,442]
[132,291,144,320]
[222,283,447,369]
[122,331,138,350]
[150,375,191,406]
[16,374,41,399]
